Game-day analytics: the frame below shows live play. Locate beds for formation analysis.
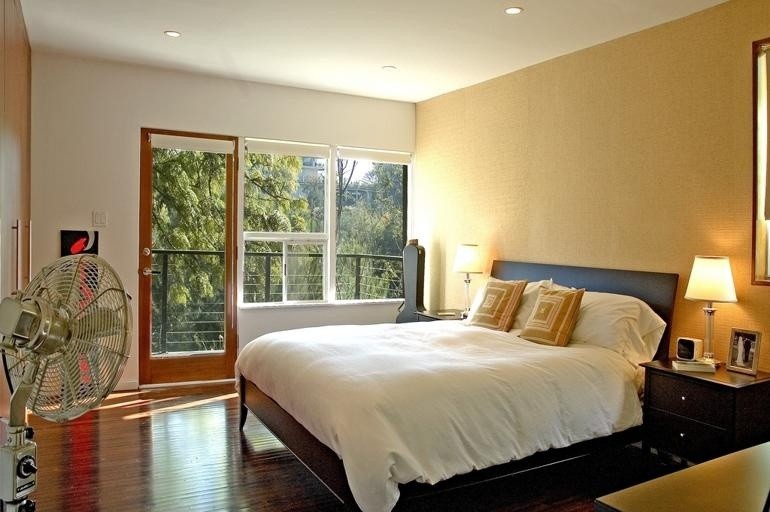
[236,260,679,510]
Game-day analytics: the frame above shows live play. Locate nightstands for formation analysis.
[639,357,770,475]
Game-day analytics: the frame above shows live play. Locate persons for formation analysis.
[743,335,751,365]
[736,336,745,366]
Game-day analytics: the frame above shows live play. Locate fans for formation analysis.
[0,253,136,511]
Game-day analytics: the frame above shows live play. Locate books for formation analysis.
[672,359,717,373]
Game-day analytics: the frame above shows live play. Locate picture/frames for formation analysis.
[726,327,762,377]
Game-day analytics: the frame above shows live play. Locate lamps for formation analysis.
[452,242,484,316]
[684,255,740,368]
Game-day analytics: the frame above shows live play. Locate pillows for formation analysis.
[465,276,668,367]
